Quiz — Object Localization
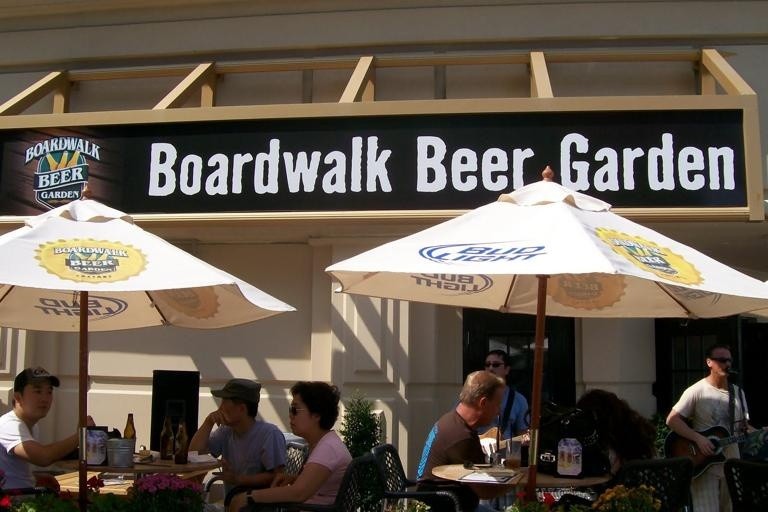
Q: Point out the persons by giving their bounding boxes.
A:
[0,365,94,499]
[475,350,528,462]
[576,389,655,461]
[224,381,352,512]
[414,371,529,512]
[188,377,288,512]
[663,346,757,512]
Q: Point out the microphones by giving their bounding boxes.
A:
[725,367,739,376]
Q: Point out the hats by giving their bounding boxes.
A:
[14,367,60,392]
[211,379,261,402]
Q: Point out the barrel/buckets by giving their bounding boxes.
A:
[105,438,136,468]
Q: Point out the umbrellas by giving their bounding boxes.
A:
[321,166,766,501]
[0,182,296,512]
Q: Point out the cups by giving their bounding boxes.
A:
[505,441,522,470]
[489,452,501,468]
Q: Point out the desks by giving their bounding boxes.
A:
[56,452,225,502]
[427,462,613,512]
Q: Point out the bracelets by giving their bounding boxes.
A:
[245,490,255,506]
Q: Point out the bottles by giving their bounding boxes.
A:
[123,413,136,439]
[175,421,190,464]
[159,411,175,461]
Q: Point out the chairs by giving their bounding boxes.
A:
[0,487,57,512]
[198,437,480,512]
[720,454,767,511]
[614,454,693,511]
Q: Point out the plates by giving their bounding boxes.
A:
[133,456,154,465]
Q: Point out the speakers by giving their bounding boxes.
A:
[150,370,200,453]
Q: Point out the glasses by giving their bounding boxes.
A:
[711,357,732,363]
[289,406,304,414]
[484,361,504,368]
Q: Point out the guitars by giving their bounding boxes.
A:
[666,426,768,479]
[475,426,529,463]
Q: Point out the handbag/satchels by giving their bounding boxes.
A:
[539,409,606,480]
